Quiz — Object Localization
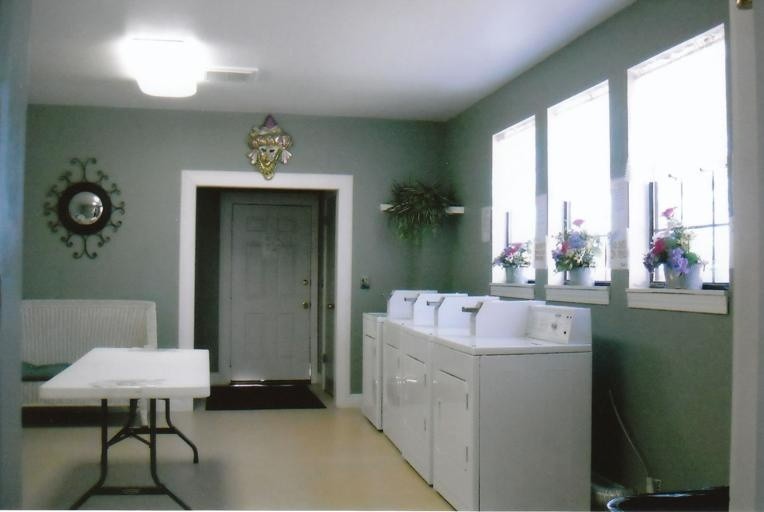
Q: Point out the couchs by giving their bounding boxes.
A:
[21,295,160,430]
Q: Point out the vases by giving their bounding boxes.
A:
[685,263,707,294]
[571,267,596,290]
[664,264,685,289]
[507,268,526,287]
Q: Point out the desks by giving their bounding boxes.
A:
[39,344,210,511]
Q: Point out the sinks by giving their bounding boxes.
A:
[605,488,728,512]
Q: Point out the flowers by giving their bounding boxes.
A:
[492,238,534,266]
[641,205,707,271]
[553,217,600,266]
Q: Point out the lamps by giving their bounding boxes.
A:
[119,37,215,100]
